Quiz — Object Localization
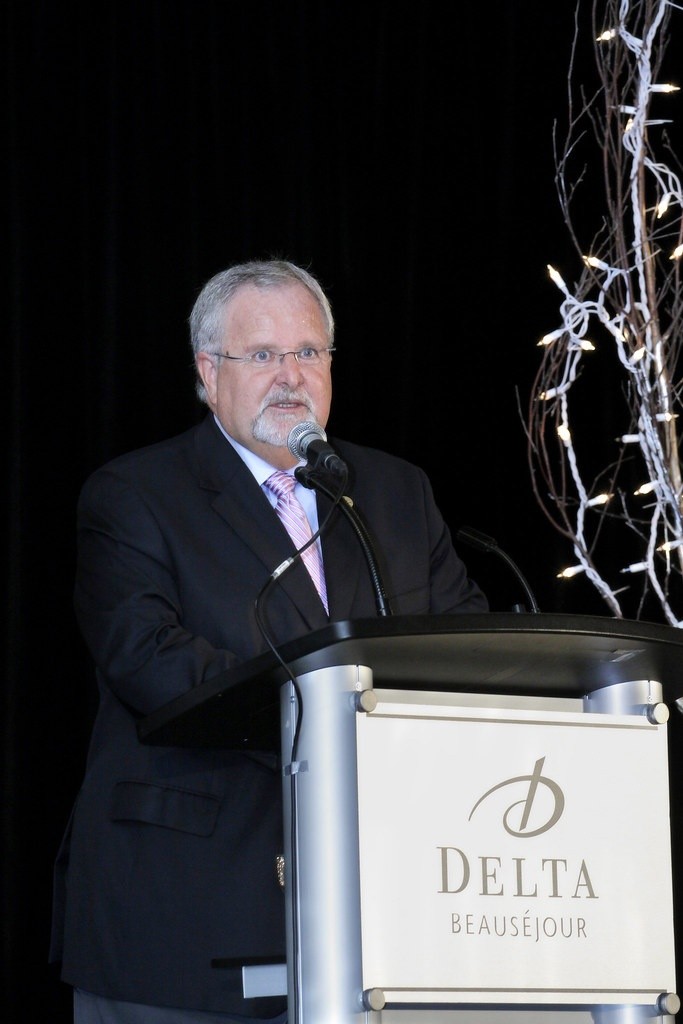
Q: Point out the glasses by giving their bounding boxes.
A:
[214,347,336,367]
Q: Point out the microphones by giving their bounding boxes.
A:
[459,527,539,615]
[287,421,347,478]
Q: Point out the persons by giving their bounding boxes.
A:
[60,260,489,1024]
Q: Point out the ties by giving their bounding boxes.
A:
[264,469,329,615]
